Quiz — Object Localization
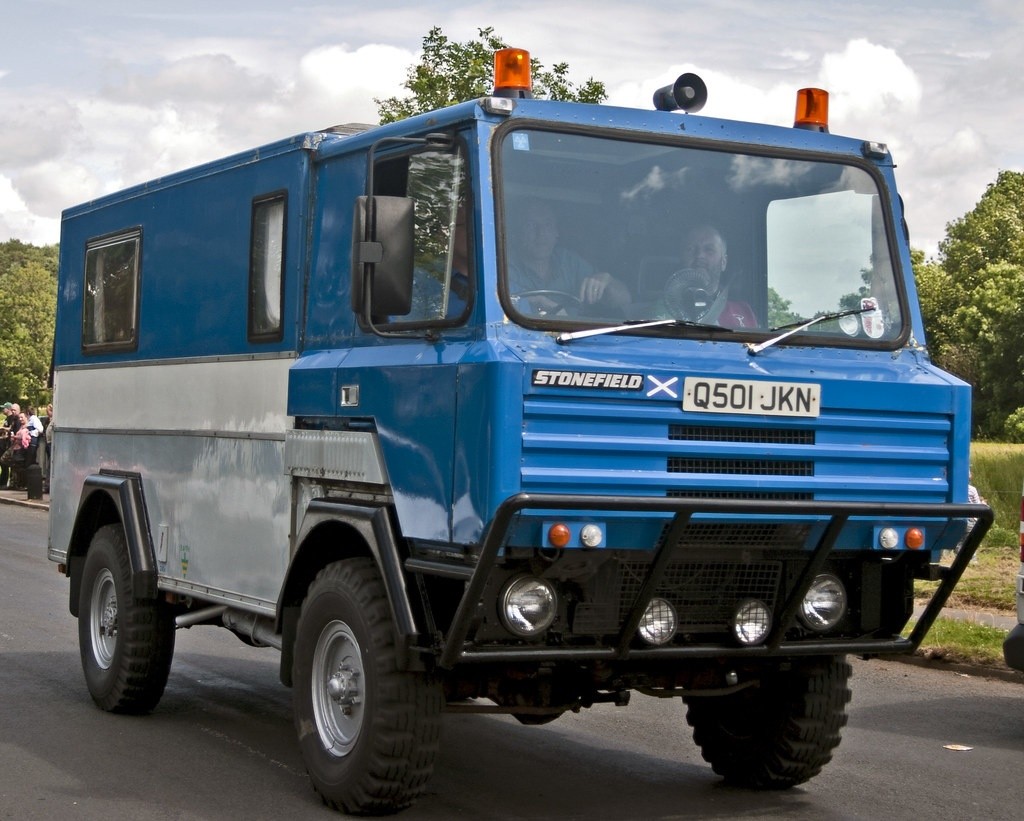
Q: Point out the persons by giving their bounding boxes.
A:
[653,223,762,330]
[388,201,535,320]
[508,198,632,320]
[0,401,53,494]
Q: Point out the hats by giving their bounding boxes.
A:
[0,402,12,410]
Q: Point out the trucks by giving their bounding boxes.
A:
[42,47,996,821]
